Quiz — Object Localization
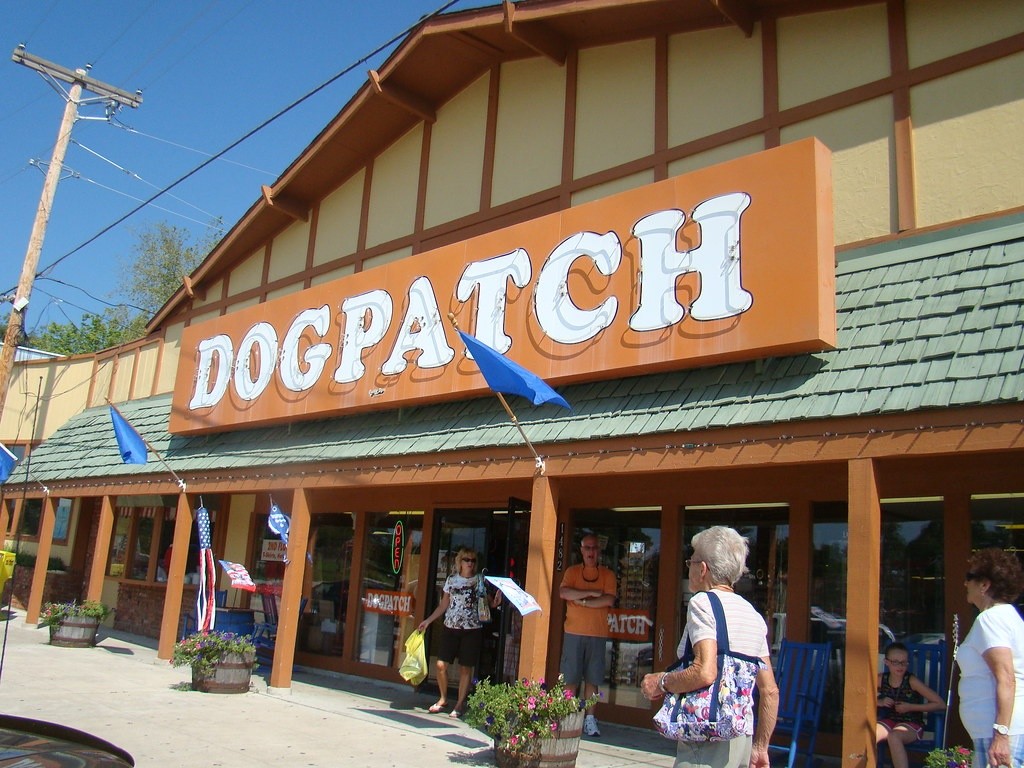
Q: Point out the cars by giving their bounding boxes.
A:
[605,616,656,677]
[806,619,945,693]
[129,538,150,576]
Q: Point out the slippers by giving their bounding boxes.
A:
[449,709,466,718]
[429,703,447,713]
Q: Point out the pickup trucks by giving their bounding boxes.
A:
[631,610,893,714]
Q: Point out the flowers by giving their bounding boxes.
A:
[924,744,975,768]
[169,630,260,679]
[41,598,109,632]
[462,674,604,755]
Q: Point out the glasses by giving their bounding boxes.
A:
[582,569,599,582]
[884,657,910,667]
[966,573,978,581]
[685,560,710,571]
[582,545,598,551]
[462,557,476,563]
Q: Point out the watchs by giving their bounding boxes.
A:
[992,723,1009,735]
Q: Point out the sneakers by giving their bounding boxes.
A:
[583,718,601,736]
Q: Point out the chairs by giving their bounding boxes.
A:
[875,639,948,768]
[248,598,309,674]
[751,637,832,768]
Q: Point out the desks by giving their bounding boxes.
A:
[215,606,269,638]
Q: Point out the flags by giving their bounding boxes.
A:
[109,405,147,464]
[0,442,19,486]
[459,331,572,411]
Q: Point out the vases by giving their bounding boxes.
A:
[493,708,585,768]
[49,616,100,647]
[191,650,256,693]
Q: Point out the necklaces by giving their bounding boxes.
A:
[891,680,902,705]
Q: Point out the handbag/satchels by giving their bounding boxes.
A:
[652,590,766,743]
[478,573,493,623]
[399,628,428,687]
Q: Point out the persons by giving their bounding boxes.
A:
[418,548,503,717]
[640,525,779,768]
[559,535,617,735]
[876,642,946,768]
[956,548,1024,768]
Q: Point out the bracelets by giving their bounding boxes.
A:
[582,598,586,607]
[658,672,670,695]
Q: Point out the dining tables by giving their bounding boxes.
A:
[181,590,228,641]
